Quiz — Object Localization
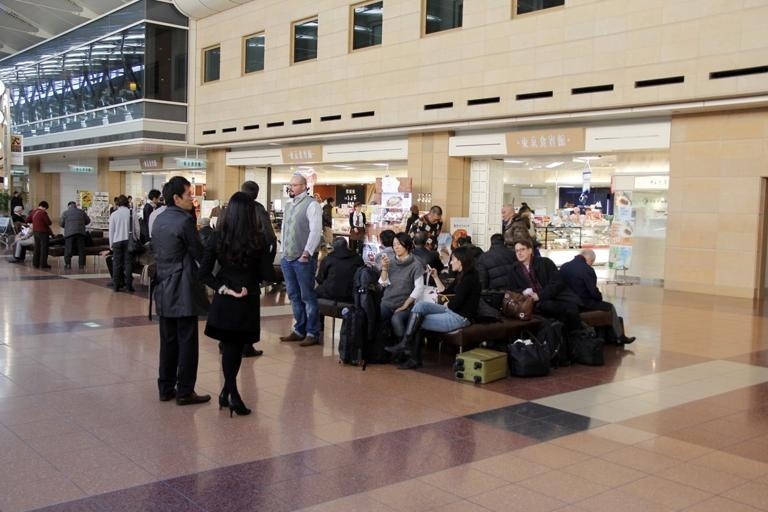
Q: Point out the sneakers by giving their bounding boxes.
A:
[279,331,305,341]
[298,335,319,347]
[243,349,264,358]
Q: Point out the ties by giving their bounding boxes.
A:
[356,215,360,227]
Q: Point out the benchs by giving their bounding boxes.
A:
[315,294,613,357]
[0,228,110,278]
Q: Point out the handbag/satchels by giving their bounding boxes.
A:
[570,328,606,367]
[438,293,457,304]
[477,323,510,351]
[534,321,570,370]
[502,288,535,320]
[422,284,440,303]
[127,232,147,257]
[507,342,549,380]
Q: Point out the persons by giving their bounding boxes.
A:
[58,201,89,270]
[148,175,211,406]
[198,191,274,419]
[312,192,635,372]
[196,205,225,320]
[218,181,276,359]
[10,190,51,270]
[278,175,322,347]
[105,188,163,293]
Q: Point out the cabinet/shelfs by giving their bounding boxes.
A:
[535,226,609,267]
[377,205,408,232]
[332,217,351,248]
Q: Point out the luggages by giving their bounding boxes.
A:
[454,348,507,385]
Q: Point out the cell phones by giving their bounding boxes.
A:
[425,264,432,272]
[383,254,388,259]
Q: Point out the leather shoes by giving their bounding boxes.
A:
[159,389,176,401]
[176,391,211,406]
[616,334,637,345]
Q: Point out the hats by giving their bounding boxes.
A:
[68,201,76,206]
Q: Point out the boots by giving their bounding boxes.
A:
[397,328,425,370]
[384,310,425,353]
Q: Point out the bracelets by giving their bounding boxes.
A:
[301,254,309,259]
[221,287,227,294]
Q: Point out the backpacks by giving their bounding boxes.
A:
[338,306,367,365]
[353,260,381,317]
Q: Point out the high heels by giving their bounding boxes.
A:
[218,391,229,411]
[229,401,252,418]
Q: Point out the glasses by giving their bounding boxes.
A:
[287,183,304,188]
[515,247,526,253]
